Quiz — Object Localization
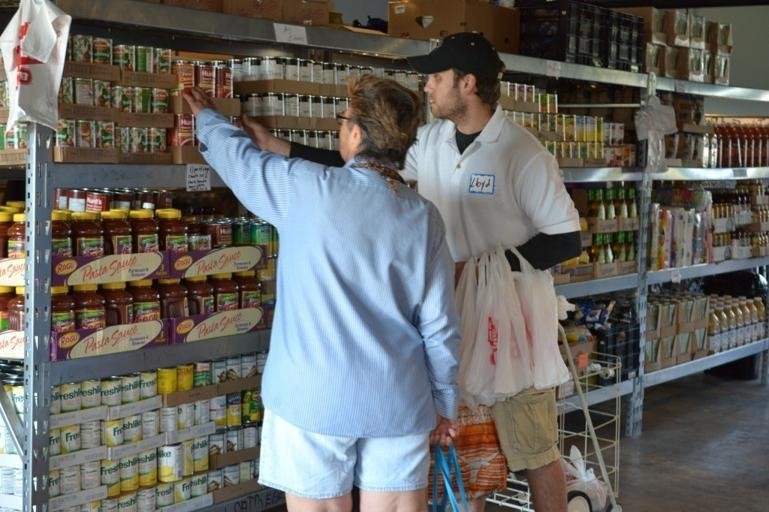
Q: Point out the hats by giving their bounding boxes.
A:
[407,32,499,79]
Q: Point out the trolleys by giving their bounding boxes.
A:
[486,320,624,512]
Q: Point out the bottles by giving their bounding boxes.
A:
[714,122,769,168]
[579,181,769,355]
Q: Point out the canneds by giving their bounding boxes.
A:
[1,181,277,335]
[0,33,638,169]
[49,350,268,512]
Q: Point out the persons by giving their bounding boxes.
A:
[182,75,460,512]
[241,32,582,512]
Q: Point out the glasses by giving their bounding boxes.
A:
[336,111,368,131]
